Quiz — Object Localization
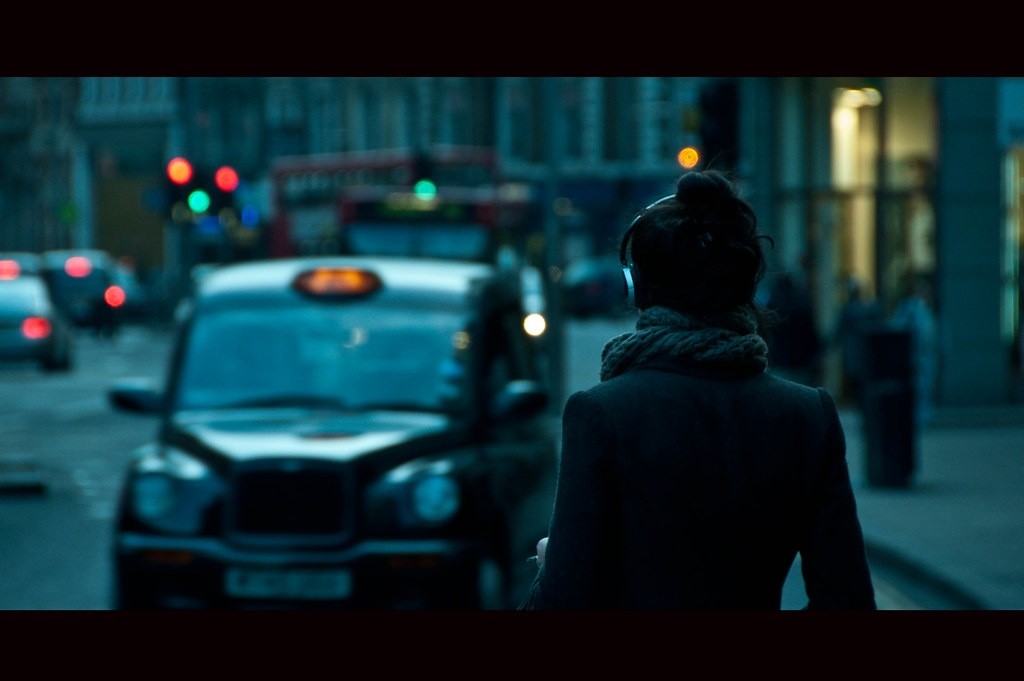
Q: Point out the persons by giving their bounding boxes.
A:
[530,169,878,612]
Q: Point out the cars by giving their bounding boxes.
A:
[0,233,134,375]
[105,250,562,629]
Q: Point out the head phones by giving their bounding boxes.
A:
[620,192,767,310]
